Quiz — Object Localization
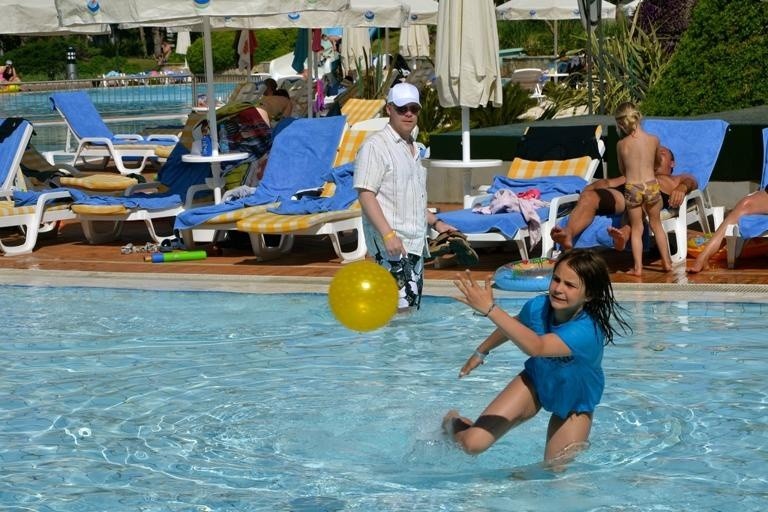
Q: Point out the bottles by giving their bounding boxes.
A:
[201,135,212,156]
[219,124,229,153]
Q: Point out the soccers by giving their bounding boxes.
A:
[329,261,400,331]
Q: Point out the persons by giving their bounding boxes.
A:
[315,45,329,68]
[354,82,462,310]
[1,60,21,84]
[551,97,768,276]
[155,42,175,66]
[379,54,413,91]
[322,33,342,54]
[255,78,295,126]
[440,251,608,471]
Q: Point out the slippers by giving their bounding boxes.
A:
[159,238,184,252]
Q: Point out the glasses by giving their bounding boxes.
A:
[391,103,419,115]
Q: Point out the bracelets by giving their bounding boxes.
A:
[382,231,399,241]
[473,349,490,359]
[470,304,499,317]
[432,218,443,228]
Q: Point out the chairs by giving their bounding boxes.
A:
[551,118,733,266]
[427,124,607,267]
[721,127,767,268]
[511,69,545,109]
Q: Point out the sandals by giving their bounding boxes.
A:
[450,230,478,268]
[428,231,452,257]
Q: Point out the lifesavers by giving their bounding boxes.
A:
[493,257,556,292]
[0,66,20,94]
[687,227,727,263]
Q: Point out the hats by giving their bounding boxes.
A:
[387,82,422,107]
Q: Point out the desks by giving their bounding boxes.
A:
[545,73,568,84]
